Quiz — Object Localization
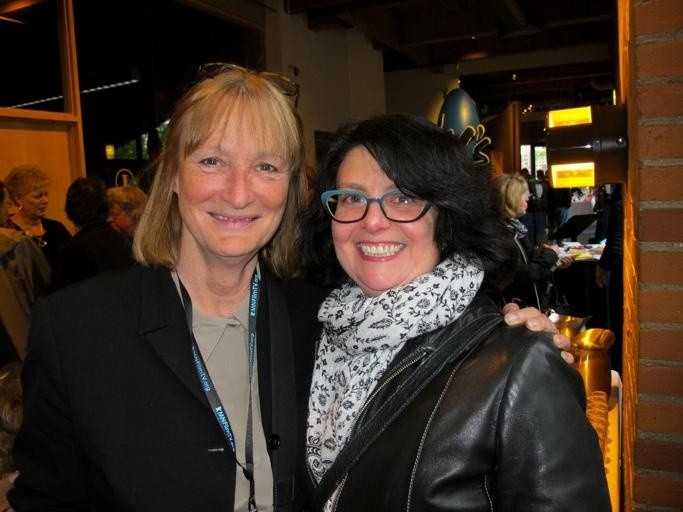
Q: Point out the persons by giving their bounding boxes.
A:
[0,167,150,361]
[278,110,616,511]
[13,57,576,512]
[493,168,605,316]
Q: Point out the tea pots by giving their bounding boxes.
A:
[544,307,615,401]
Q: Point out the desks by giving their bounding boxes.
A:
[602,370,624,512]
[548,241,607,325]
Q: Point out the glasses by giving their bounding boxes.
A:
[321,188,435,222]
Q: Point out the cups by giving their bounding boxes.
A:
[586,390,607,470]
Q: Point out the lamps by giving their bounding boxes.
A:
[545,101,628,191]
